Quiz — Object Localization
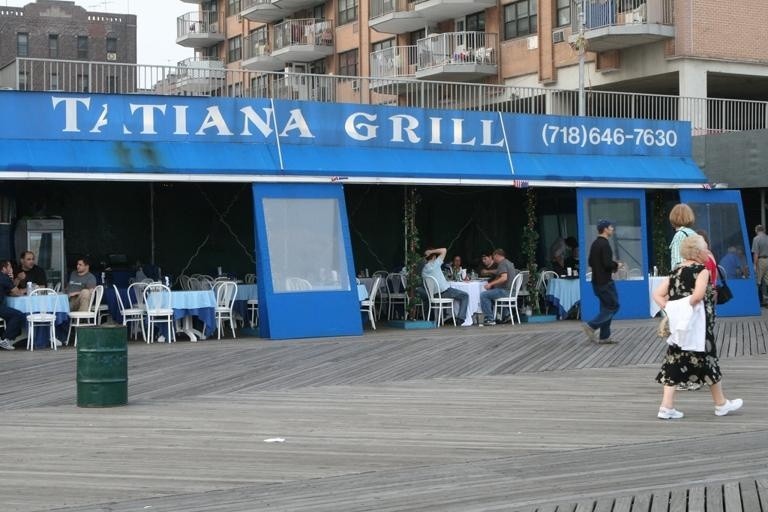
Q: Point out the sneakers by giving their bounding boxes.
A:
[581,324,596,343]
[656,405,685,420]
[484,320,496,326]
[501,314,513,324]
[713,396,745,416]
[0,337,15,350]
[598,336,616,345]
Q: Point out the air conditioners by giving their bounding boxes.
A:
[210,24,217,32]
[106,53,116,61]
[552,29,566,44]
[235,14,242,23]
[352,80,360,90]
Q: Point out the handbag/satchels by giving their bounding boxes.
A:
[716,282,733,305]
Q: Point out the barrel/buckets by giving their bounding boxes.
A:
[75,323,129,409]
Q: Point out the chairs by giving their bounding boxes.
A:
[625,3,647,23]
[474,46,493,65]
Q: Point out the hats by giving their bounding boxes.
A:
[597,219,616,230]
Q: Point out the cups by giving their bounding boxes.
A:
[47,283,53,294]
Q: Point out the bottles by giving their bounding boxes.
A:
[444,266,479,280]
[26,281,45,296]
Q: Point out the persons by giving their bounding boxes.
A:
[0,258,21,350]
[15,250,46,294]
[669,204,706,269]
[653,236,743,419]
[62,257,97,311]
[583,219,623,344]
[417,237,578,326]
[718,222,767,308]
